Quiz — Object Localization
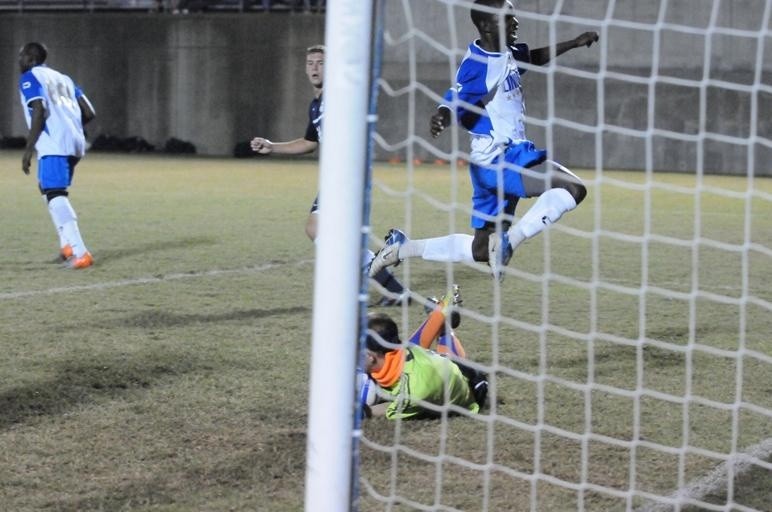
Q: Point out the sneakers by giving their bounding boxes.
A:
[50,244,93,268]
[368,229,406,279]
[488,230,512,280]
[368,289,412,307]
[424,285,461,336]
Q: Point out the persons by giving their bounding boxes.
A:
[354,284,489,421]
[367,0,599,283]
[249,45,411,309]
[16,41,97,270]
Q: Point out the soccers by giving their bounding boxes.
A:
[358,369,381,407]
